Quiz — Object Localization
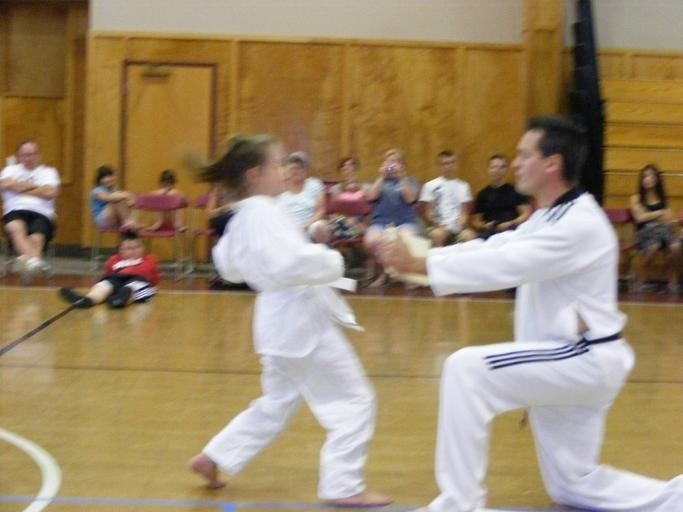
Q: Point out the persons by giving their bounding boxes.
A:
[0,140,61,279]
[147,170,184,230]
[626,163,682,297]
[86,165,143,237]
[206,148,535,299]
[57,228,161,311]
[179,130,390,507]
[370,114,682,511]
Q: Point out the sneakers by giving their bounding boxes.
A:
[8,257,54,283]
[61,288,91,308]
[108,287,132,308]
